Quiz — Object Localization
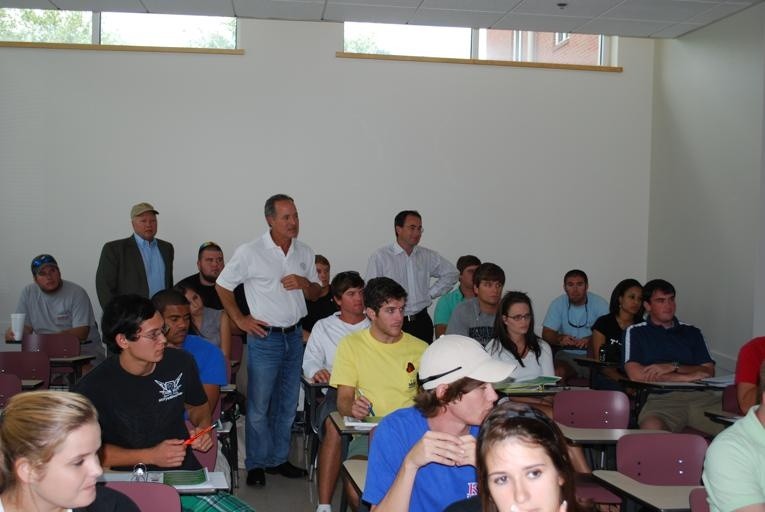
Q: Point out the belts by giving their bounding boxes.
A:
[257,320,302,333]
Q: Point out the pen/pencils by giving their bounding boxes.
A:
[356,389,375,417]
[184,424,218,444]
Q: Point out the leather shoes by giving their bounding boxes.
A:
[265,460,308,478]
[247,468,265,487]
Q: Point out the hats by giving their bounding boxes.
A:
[130,201,159,219]
[418,334,518,391]
[30,254,58,274]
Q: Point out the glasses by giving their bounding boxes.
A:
[403,223,424,233]
[139,325,170,340]
[417,367,462,388]
[568,314,588,328]
[507,313,531,321]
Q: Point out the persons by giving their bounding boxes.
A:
[301,255,764,512]
[0,242,249,511]
[362,208,462,345]
[95,201,174,359]
[215,194,324,489]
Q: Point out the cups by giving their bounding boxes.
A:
[10,313,26,341]
